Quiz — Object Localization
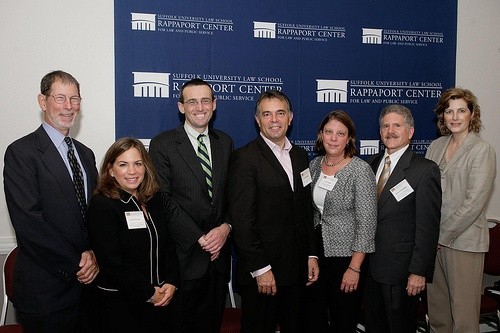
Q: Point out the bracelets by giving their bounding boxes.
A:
[349,265,360,273]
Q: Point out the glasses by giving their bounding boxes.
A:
[182,97,215,106]
[46,93,84,106]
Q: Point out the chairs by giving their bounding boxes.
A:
[0,246,22,333]
[478,218,500,333]
[220,257,242,333]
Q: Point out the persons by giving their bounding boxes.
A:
[228,91,320,333]
[309,109,377,333]
[150,79,235,333]
[85,136,182,333]
[425,89,497,333]
[362,104,440,333]
[3,70,100,333]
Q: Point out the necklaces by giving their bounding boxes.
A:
[324,153,345,167]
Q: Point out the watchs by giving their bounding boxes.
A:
[224,222,232,231]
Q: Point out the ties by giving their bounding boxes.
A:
[65,136,88,220]
[197,135,212,206]
[377,156,391,203]
